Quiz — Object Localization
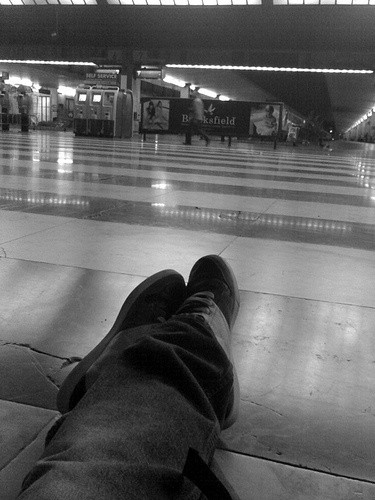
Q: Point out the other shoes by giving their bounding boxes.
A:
[56,268,187,415]
[178,254,242,431]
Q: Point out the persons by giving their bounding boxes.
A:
[183,91,212,147]
[16,254,244,500]
[256,106,278,138]
[142,100,171,131]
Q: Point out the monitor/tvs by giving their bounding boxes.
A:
[92,93,101,103]
[78,93,87,102]
